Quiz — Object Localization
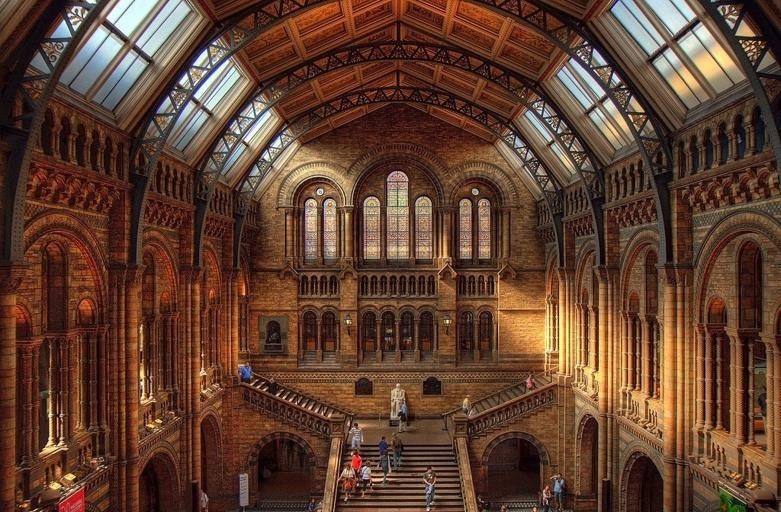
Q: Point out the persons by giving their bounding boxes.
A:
[423,465,437,512]
[399,401,411,427]
[526,373,538,391]
[200,489,210,511]
[283,439,296,465]
[550,472,565,511]
[757,390,768,436]
[462,394,473,416]
[397,409,407,433]
[334,422,406,501]
[241,361,253,384]
[308,499,316,512]
[390,382,407,417]
[265,378,278,395]
[476,484,553,511]
[469,405,478,434]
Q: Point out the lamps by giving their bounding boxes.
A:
[443,314,453,335]
[344,314,353,336]
